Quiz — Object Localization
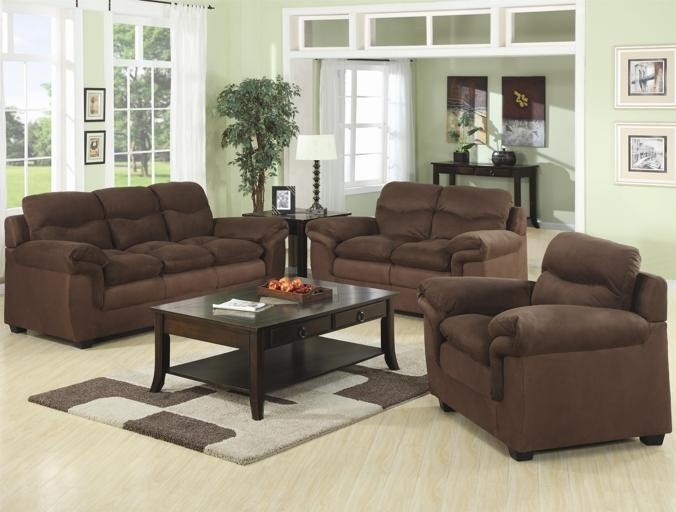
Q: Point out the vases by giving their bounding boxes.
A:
[492,151,516,165]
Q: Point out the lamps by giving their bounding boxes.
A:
[296,135,338,215]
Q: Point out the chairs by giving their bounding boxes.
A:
[417,270,676,462]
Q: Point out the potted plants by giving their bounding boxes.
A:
[447,110,482,163]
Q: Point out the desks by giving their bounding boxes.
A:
[431,162,540,229]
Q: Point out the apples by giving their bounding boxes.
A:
[268,276,303,292]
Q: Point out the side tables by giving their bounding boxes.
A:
[242,208,352,278]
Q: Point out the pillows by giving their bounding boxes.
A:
[430,185,513,240]
[375,181,444,240]
[21,182,214,251]
[530,231,641,311]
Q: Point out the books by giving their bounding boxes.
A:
[213,309,257,319]
[213,298,272,313]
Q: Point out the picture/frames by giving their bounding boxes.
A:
[614,44,675,108]
[84,88,106,122]
[84,131,106,165]
[272,186,295,215]
[615,122,675,187]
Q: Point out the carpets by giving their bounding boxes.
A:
[27,329,430,467]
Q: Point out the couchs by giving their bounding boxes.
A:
[3,182,290,347]
[305,182,528,315]
